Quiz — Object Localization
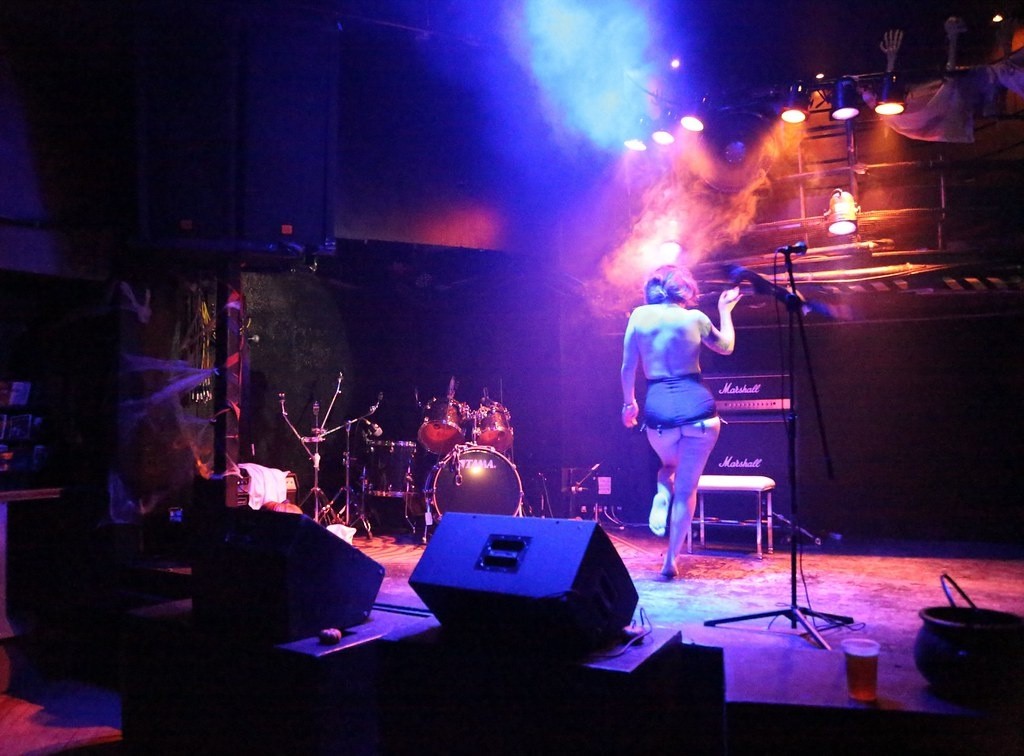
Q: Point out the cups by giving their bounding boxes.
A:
[841,637,881,702]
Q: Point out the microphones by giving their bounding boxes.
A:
[777,242,807,256]
[571,486,588,492]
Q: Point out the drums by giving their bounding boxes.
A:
[361,439,417,498]
[473,402,513,454]
[424,444,523,524]
[417,396,467,457]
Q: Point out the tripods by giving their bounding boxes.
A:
[704,254,854,650]
[298,401,372,539]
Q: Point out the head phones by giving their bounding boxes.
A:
[646,274,674,306]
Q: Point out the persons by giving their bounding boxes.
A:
[424,368,472,481]
[620,262,743,576]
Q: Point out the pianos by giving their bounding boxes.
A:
[700,371,821,546]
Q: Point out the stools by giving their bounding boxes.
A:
[686,474,776,559]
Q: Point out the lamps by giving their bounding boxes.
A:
[825,187,862,235]
[622,72,910,150]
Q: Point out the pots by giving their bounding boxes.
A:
[913,572,1024,710]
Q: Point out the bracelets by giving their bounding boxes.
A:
[624,398,636,409]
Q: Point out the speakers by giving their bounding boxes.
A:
[192,509,387,646]
[407,510,638,646]
[691,415,800,532]
